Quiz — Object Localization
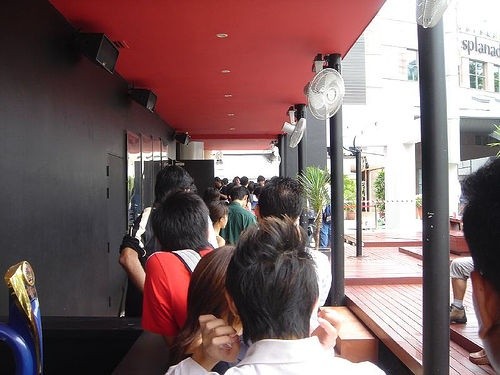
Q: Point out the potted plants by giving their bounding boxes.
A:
[343,174,356,220]
[373,168,385,221]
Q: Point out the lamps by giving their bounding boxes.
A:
[76,32,191,145]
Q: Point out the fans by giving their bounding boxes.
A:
[281,106,306,148]
[303,53,345,120]
[266,143,281,164]
[416,0,451,29]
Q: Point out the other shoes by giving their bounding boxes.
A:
[469,349,490,365]
[450,303,467,323]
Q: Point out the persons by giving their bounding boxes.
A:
[141,189,215,350]
[449,257,475,324]
[205,175,270,247]
[460,156,500,375]
[158,246,252,375]
[319,183,332,247]
[255,175,332,307]
[166,217,386,375]
[118,165,194,316]
[207,201,230,247]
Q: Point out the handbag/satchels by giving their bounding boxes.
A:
[323,211,332,224]
